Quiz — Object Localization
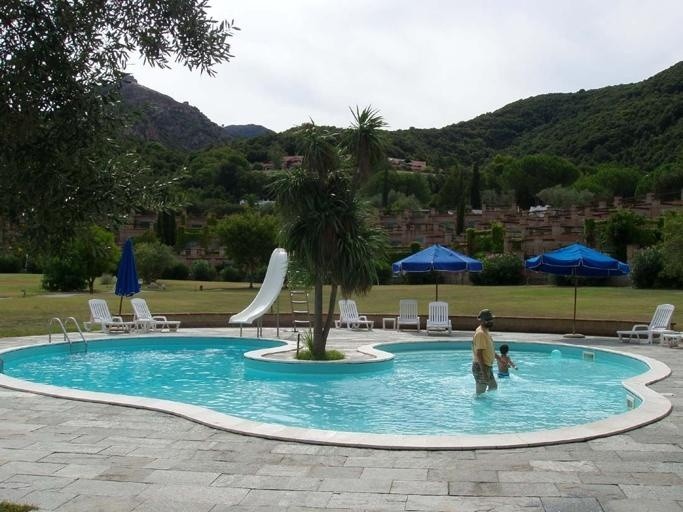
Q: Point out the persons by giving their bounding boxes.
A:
[498,344,518,378]
[472,309,507,394]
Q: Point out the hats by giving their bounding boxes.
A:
[478,308,495,321]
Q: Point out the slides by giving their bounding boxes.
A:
[228,248,295,324]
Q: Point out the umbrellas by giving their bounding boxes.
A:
[526,242,631,334]
[114,237,141,314]
[392,243,483,302]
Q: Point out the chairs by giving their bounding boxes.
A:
[131,298,181,333]
[83,297,150,336]
[426,302,452,336]
[335,300,374,332]
[396,299,420,333]
[662,333,683,349]
[616,304,675,344]
